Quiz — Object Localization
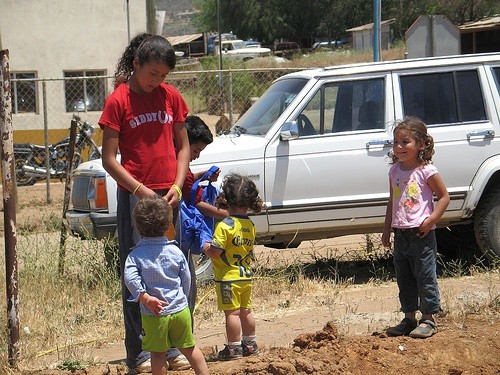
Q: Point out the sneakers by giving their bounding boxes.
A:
[241,339,260,357]
[167,353,192,371]
[217,343,243,361]
[129,358,169,373]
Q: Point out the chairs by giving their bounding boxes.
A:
[357,102,380,128]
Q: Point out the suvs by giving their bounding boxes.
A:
[64,50,500,271]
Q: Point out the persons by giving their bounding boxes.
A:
[382,117,450,338]
[174,115,262,360]
[123,196,208,375]
[97,32,209,373]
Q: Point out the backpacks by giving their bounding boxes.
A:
[190,165,222,232]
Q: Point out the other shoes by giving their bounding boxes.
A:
[408,318,438,338]
[387,318,419,336]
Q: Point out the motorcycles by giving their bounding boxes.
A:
[13,106,119,187]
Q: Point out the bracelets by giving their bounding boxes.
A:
[171,184,183,200]
[133,182,143,195]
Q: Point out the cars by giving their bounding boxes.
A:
[71,93,93,112]
[207,30,343,82]
